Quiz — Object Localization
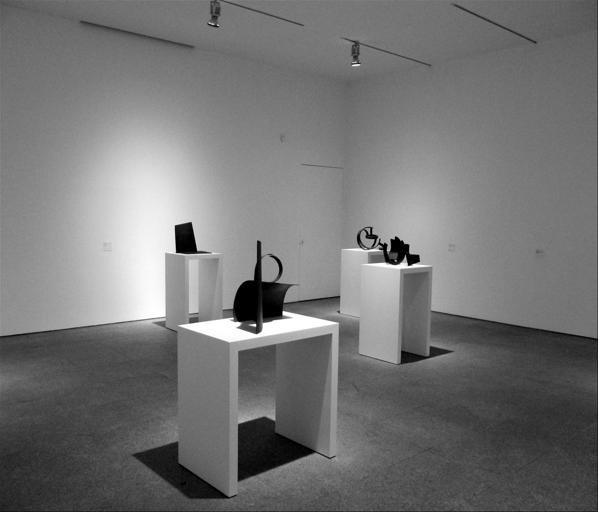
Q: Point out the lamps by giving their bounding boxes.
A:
[205,0,222,29]
[350,41,362,68]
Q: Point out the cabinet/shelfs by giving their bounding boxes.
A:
[163,250,224,331]
[358,262,435,366]
[338,248,390,317]
[175,308,340,499]
